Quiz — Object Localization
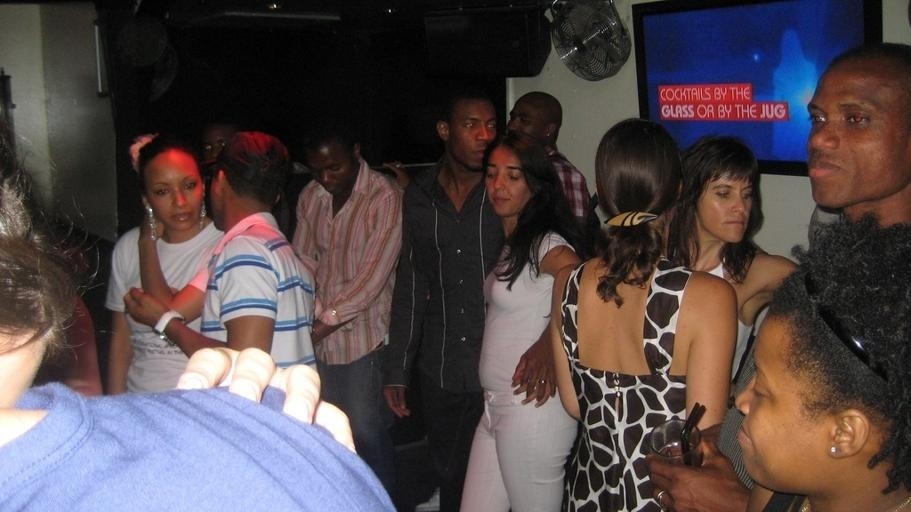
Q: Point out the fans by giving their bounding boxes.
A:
[551,0,632,82]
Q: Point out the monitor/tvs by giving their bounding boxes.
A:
[631,0,884,178]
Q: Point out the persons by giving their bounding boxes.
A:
[3,40,911,511]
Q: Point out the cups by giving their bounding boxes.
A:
[648,418,704,468]
